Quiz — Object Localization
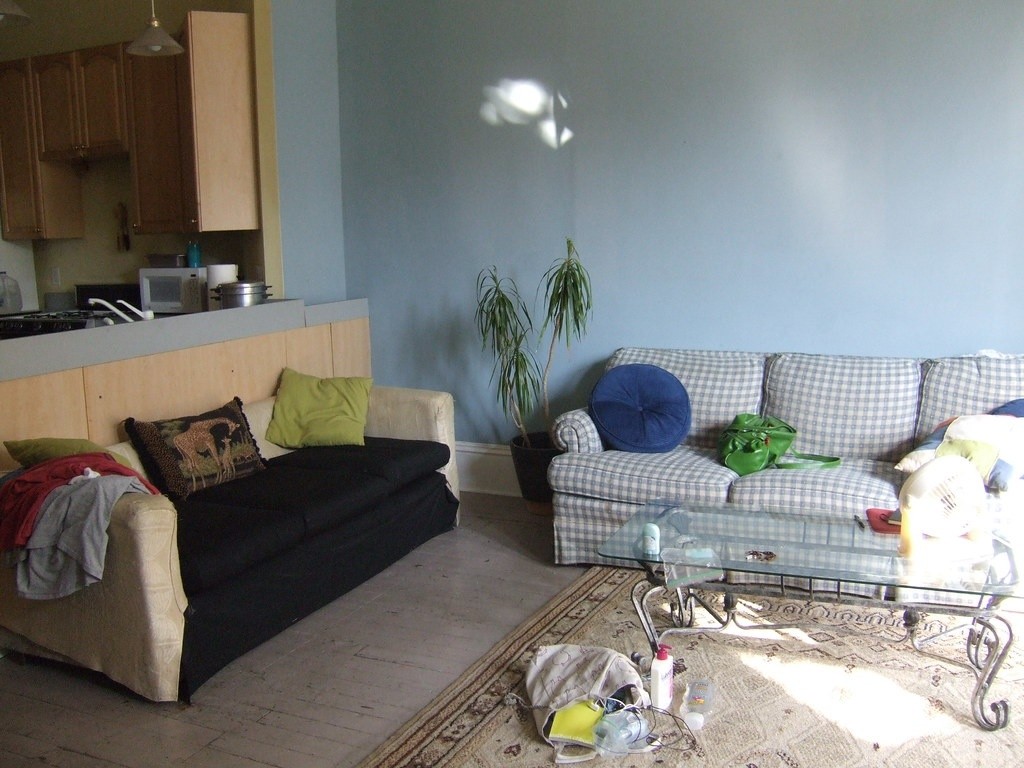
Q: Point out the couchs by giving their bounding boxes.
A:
[1,383,459,702]
[547,345,1024,602]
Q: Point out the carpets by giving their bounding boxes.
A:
[353,568,1024,768]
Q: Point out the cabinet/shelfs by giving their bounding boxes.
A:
[34,42,125,161]
[129,35,187,236]
[0,58,85,241]
[175,11,256,235]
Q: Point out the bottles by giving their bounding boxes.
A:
[680,680,712,731]
[0,271,23,314]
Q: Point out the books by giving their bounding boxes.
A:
[548,699,605,764]
[866,509,902,534]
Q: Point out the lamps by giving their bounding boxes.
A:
[126,0,186,57]
[0,0,34,27]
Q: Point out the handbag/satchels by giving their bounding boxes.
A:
[718,413,842,477]
[524,643,664,763]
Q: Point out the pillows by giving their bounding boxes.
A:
[587,363,693,452]
[2,438,130,473]
[266,368,374,447]
[124,398,267,505]
[894,412,1024,491]
[988,399,1024,416]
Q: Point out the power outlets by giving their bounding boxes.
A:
[51,267,61,286]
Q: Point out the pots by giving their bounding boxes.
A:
[210,274,274,309]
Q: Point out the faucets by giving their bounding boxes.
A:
[88,298,154,322]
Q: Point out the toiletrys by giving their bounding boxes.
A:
[679,681,715,730]
[651,643,674,711]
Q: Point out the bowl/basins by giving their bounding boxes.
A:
[144,254,187,269]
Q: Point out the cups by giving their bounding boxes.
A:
[207,264,239,311]
[187,244,201,268]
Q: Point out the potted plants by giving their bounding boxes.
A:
[475,235,593,516]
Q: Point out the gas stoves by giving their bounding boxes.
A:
[0,283,143,340]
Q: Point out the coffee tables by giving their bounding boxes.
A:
[599,498,1024,732]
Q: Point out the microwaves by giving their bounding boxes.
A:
[139,267,207,313]
